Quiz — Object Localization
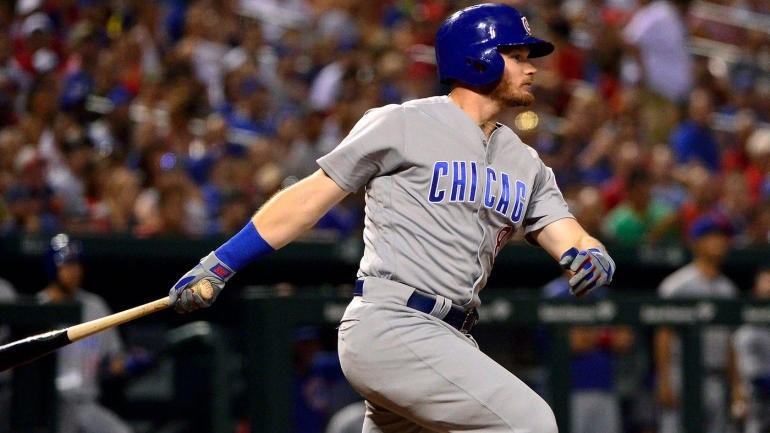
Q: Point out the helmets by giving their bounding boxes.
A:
[435,2,557,95]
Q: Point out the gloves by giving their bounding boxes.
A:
[559,246,615,301]
[167,250,236,313]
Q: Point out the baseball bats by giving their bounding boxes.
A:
[0,280,216,372]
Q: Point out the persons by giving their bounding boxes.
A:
[169,3,616,433]
[0,1,770,433]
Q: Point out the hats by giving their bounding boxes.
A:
[51,236,82,265]
[691,211,733,239]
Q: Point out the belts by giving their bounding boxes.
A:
[354,281,478,336]
